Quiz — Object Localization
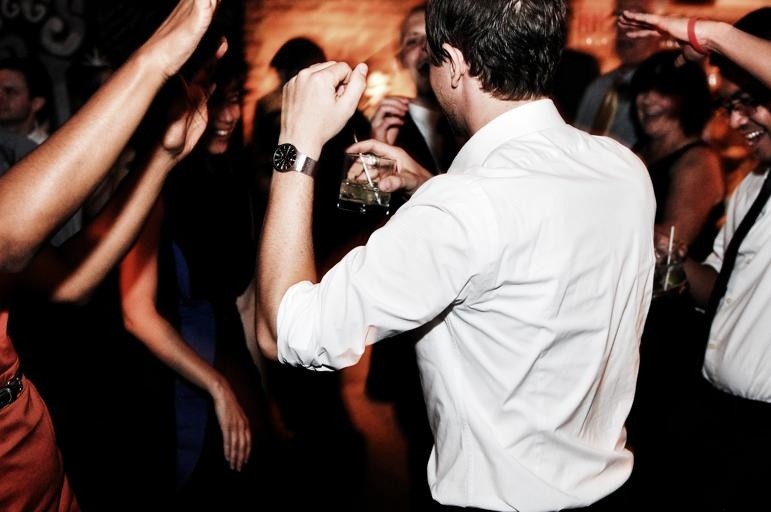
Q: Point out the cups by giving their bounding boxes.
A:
[337,153,396,219]
[652,240,687,299]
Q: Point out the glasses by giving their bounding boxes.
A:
[720,100,757,118]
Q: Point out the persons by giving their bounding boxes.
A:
[2,1,771,511]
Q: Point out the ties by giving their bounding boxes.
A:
[701,168,771,339]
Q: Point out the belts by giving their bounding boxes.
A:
[0,369,22,408]
